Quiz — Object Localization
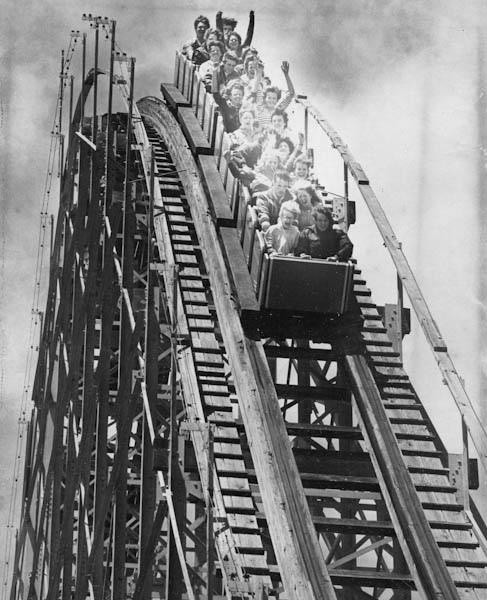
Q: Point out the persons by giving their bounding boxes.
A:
[180,9,350,263]
